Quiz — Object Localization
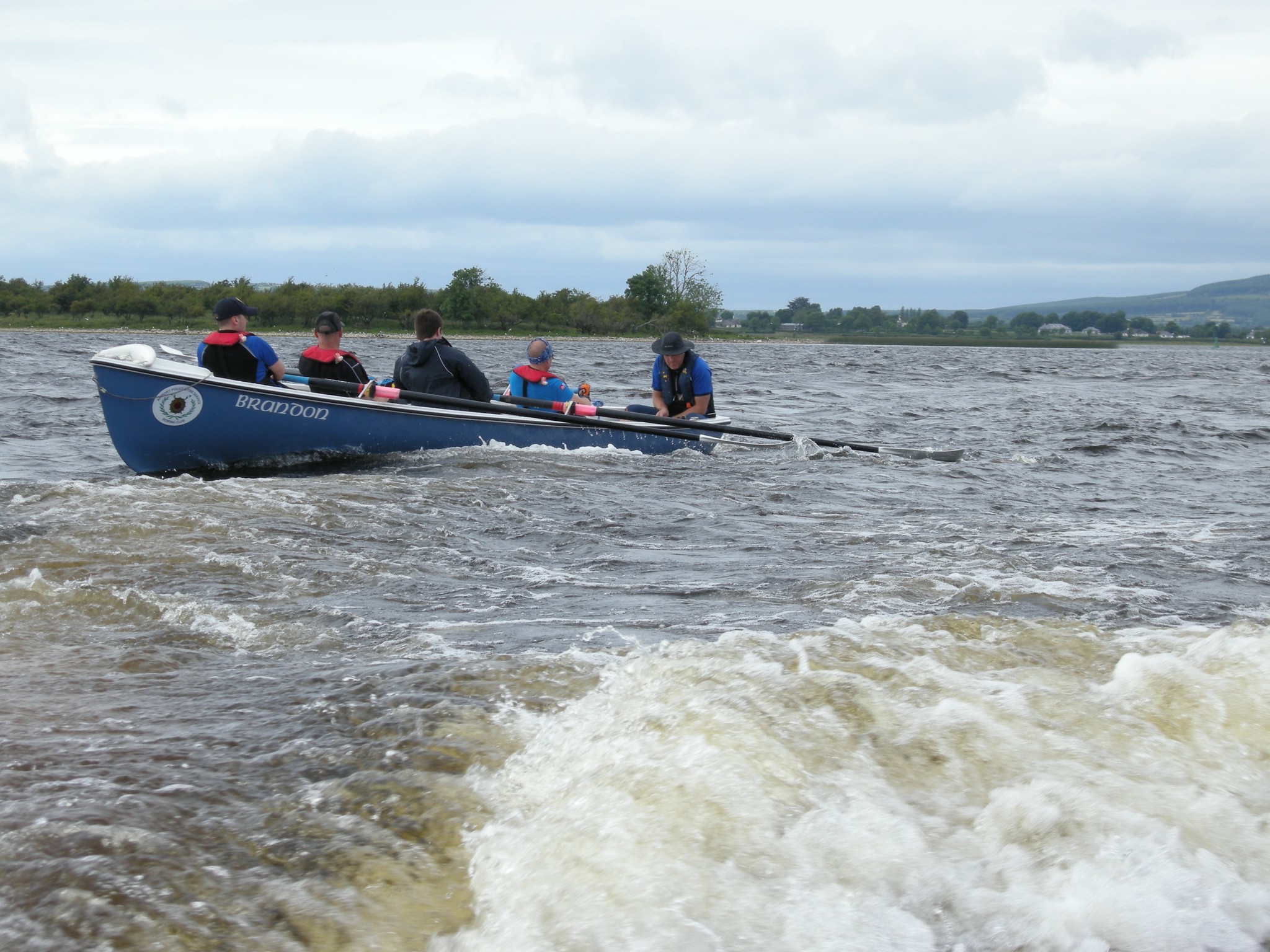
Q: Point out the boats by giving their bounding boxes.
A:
[88,342,733,483]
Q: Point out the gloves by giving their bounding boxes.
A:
[578,384,592,401]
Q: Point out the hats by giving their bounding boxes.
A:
[315,311,344,334]
[651,332,695,355]
[213,297,257,320]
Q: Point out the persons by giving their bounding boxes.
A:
[394,310,500,414]
[298,312,407,404]
[625,332,716,419]
[509,338,591,418]
[197,297,296,390]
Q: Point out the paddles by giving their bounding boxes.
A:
[491,395,965,462]
[160,344,302,376]
[282,373,792,448]
